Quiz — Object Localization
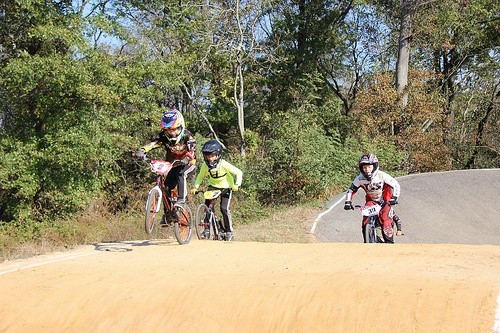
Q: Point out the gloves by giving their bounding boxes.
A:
[181,156,190,166]
[232,184,241,192]
[344,201,354,210]
[388,196,397,205]
[190,186,200,196]
[135,149,145,160]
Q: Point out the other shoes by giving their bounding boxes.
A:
[200,229,209,239]
[226,234,234,241]
[174,199,184,209]
[160,215,165,224]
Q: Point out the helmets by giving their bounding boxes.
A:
[359,153,379,181]
[202,140,222,168]
[162,109,185,145]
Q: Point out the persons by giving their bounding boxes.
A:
[135,109,197,227]
[190,140,243,242]
[345,153,401,244]
